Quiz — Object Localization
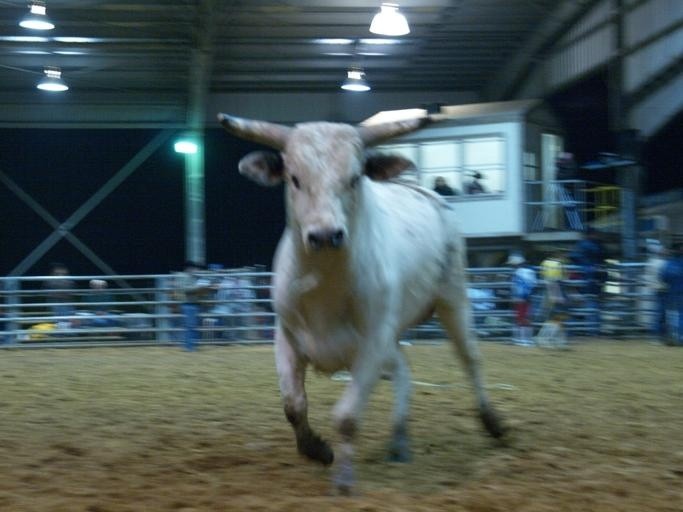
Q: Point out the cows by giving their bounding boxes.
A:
[214,109,508,469]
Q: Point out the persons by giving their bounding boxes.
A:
[16,259,275,353]
[468,226,683,350]
[433,177,456,196]
[469,173,485,193]
[554,152,581,191]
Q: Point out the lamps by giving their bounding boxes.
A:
[18,1,55,32]
[37,65,70,92]
[369,1,410,39]
[340,69,371,95]
